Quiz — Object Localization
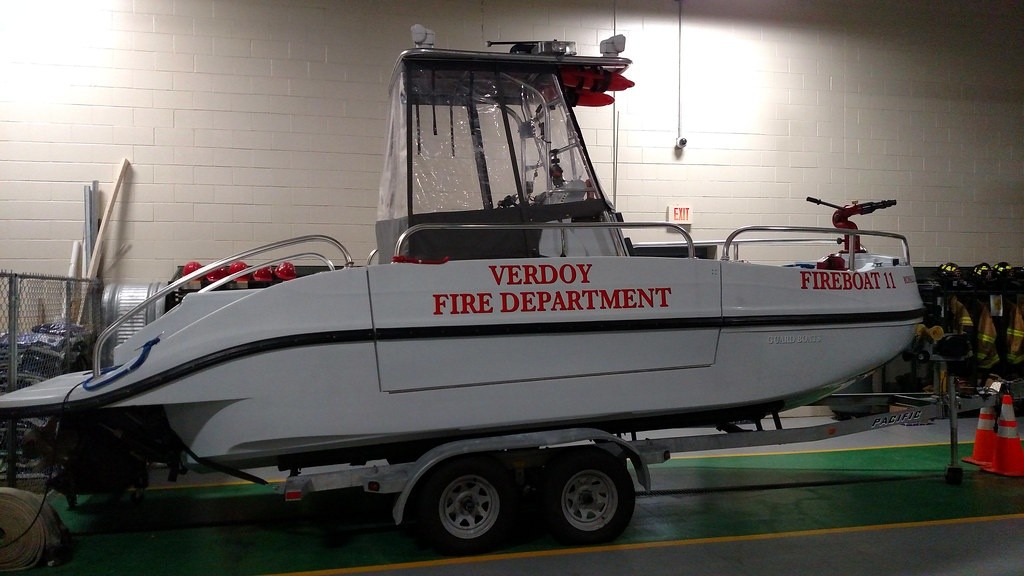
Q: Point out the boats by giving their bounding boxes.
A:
[0,23,925,464]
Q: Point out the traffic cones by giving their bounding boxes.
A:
[961,406,997,466]
[982,395,1024,477]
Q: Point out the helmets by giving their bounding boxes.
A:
[973,262,993,279]
[1009,267,1024,278]
[936,262,963,280]
[993,261,1013,278]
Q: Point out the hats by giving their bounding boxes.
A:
[274,262,296,280]
[253,266,274,282]
[205,266,228,284]
[228,262,252,281]
[182,261,205,281]
